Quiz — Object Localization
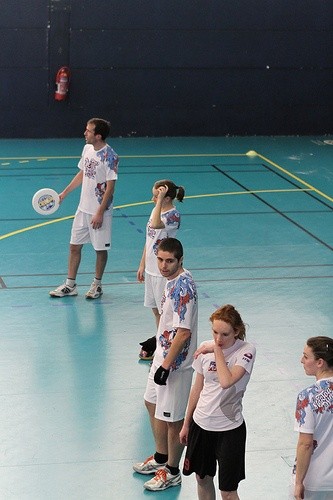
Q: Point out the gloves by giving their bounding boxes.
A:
[138,336,156,355]
[152,366,170,386]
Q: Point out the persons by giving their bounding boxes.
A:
[137,180,185,360]
[132,238,198,491]
[294,336,333,500]
[179,304,256,500]
[49,118,119,300]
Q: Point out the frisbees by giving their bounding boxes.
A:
[32,188,60,216]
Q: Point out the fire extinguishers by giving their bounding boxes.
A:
[53,65,72,102]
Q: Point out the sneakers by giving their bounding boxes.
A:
[132,454,167,474]
[84,283,102,298]
[143,466,182,490]
[48,284,77,297]
